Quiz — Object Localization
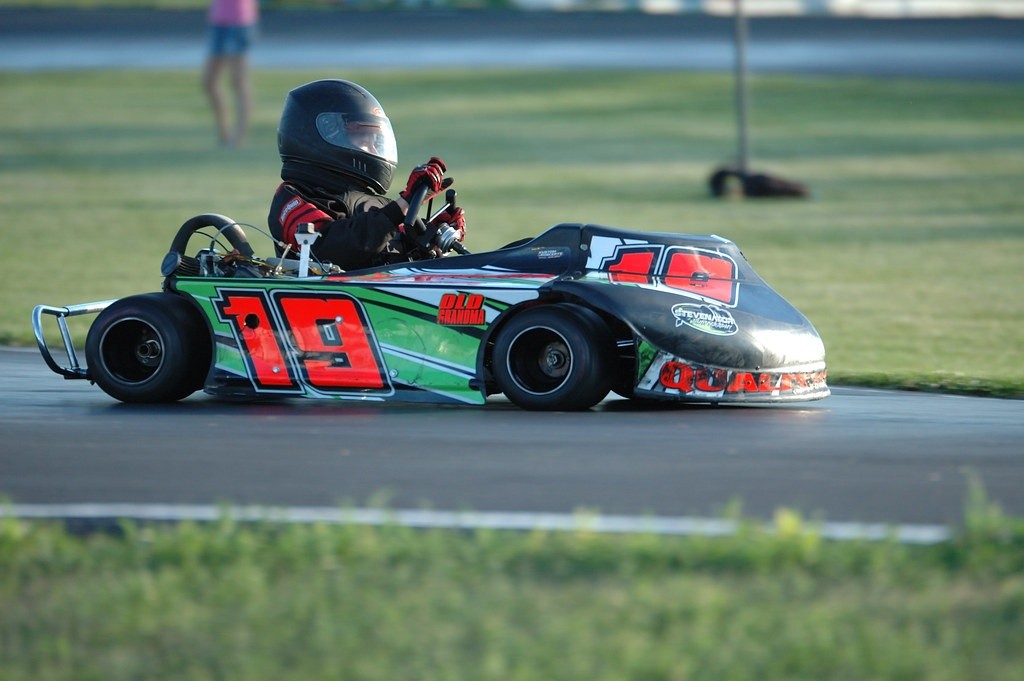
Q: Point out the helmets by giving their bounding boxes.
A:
[276,79,398,220]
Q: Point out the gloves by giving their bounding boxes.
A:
[430,207,466,243]
[399,157,454,207]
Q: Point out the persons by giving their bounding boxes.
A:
[267,79,466,269]
[201,0,259,148]
